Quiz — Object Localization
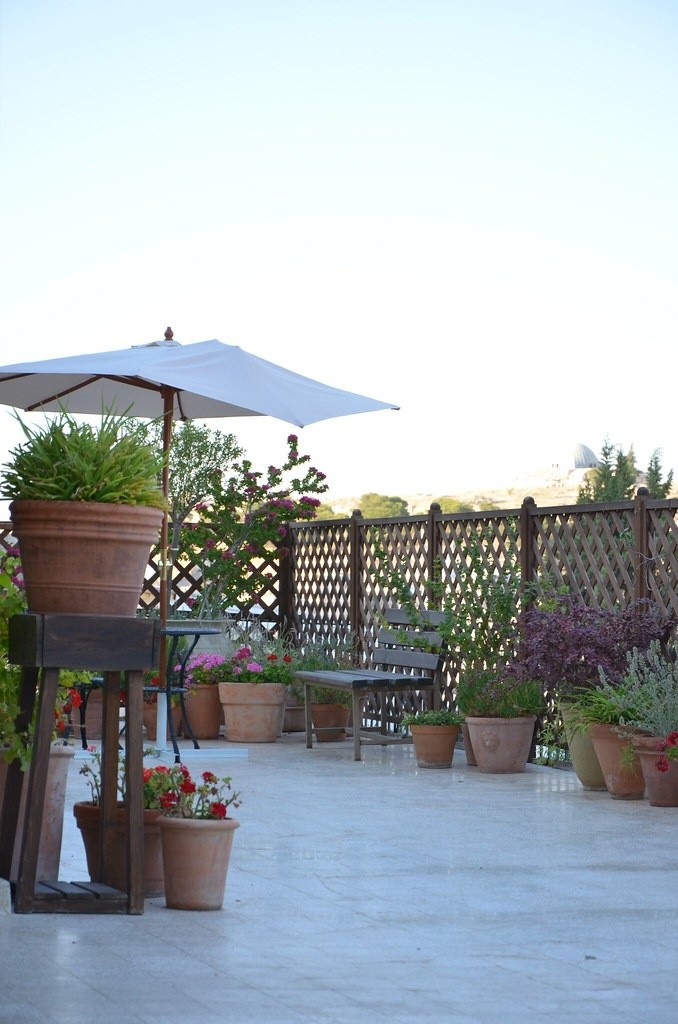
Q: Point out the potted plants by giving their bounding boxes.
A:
[455,668,543,774]
[366,517,539,766]
[142,669,183,741]
[173,651,223,738]
[282,685,306,732]
[348,695,369,728]
[0,593,93,883]
[0,394,179,618]
[597,638,678,807]
[400,709,463,769]
[295,651,352,741]
[218,648,292,742]
[153,762,242,911]
[57,670,103,739]
[502,589,674,792]
[568,685,645,800]
[72,742,178,898]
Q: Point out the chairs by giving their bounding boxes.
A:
[55,677,123,752]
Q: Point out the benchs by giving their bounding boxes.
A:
[294,608,453,760]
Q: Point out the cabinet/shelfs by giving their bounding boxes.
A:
[9,613,163,913]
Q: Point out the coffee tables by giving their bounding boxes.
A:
[119,625,222,764]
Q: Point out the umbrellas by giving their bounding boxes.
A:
[0,325,402,693]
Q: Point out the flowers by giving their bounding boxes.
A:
[143,763,241,820]
[79,745,156,807]
[174,652,227,698]
[181,435,329,609]
[54,688,83,732]
[656,730,678,772]
[0,545,25,599]
[220,646,292,683]
[118,667,160,704]
